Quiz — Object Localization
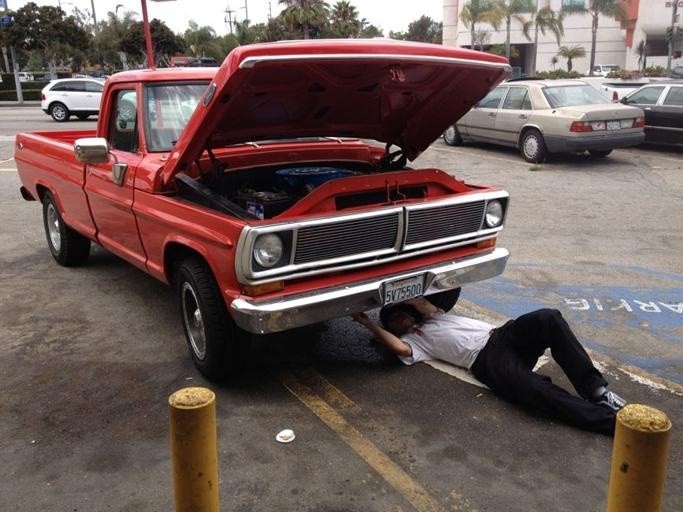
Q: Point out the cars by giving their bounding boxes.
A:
[584,62,682,158]
[439,78,647,165]
[15,70,109,83]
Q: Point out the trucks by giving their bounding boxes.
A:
[168,54,219,67]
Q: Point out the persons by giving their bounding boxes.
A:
[349,294,624,437]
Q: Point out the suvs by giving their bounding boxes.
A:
[39,77,159,123]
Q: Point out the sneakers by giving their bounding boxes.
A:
[589,391,627,412]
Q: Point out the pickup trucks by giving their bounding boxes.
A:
[12,36,517,382]
